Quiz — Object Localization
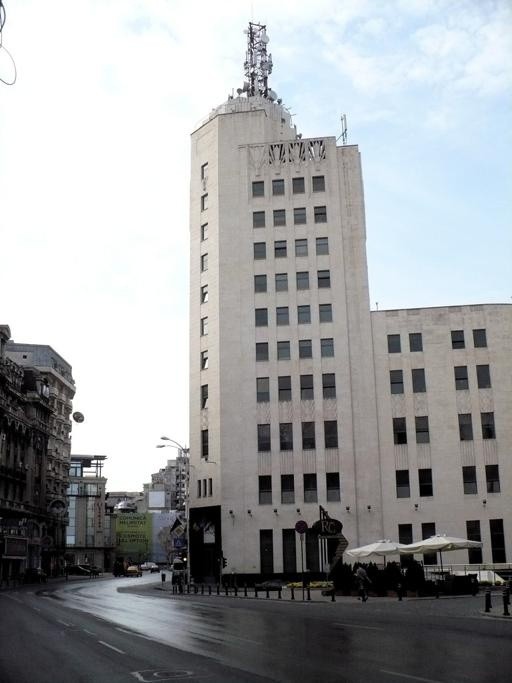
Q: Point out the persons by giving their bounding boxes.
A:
[351,562,372,601]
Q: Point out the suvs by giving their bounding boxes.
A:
[24,567,48,583]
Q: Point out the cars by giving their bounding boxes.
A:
[66,563,104,575]
[112,556,160,578]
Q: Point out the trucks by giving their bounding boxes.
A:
[168,556,190,585]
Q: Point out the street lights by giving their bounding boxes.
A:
[155,435,193,584]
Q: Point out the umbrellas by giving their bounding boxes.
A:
[345,538,406,568]
[397,533,482,580]
